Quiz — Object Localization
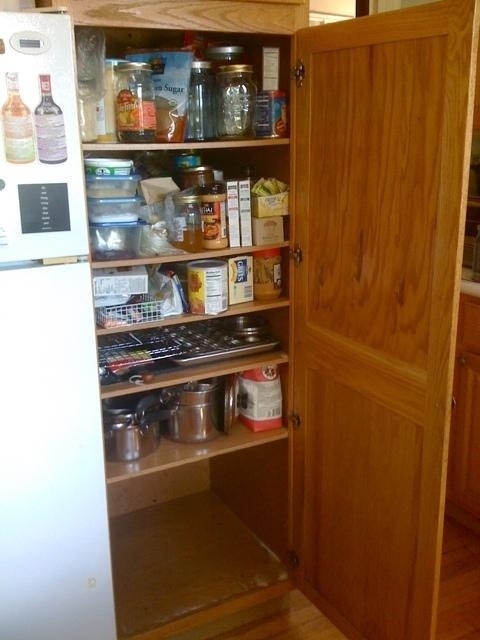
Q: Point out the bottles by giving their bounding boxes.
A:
[0,70,35,166]
[186,61,217,139]
[217,63,258,140]
[207,45,247,63]
[34,72,69,166]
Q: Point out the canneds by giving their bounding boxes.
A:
[181,166,213,189]
[171,194,204,253]
[254,249,283,300]
[200,185,229,250]
[254,90,289,139]
[185,259,228,316]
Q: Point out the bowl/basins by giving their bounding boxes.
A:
[224,316,271,344]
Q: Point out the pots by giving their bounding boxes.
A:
[103,371,239,464]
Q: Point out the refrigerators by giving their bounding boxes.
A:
[0,10,117,639]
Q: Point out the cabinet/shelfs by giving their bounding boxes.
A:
[443,159,479,543]
[33,0,479,640]
